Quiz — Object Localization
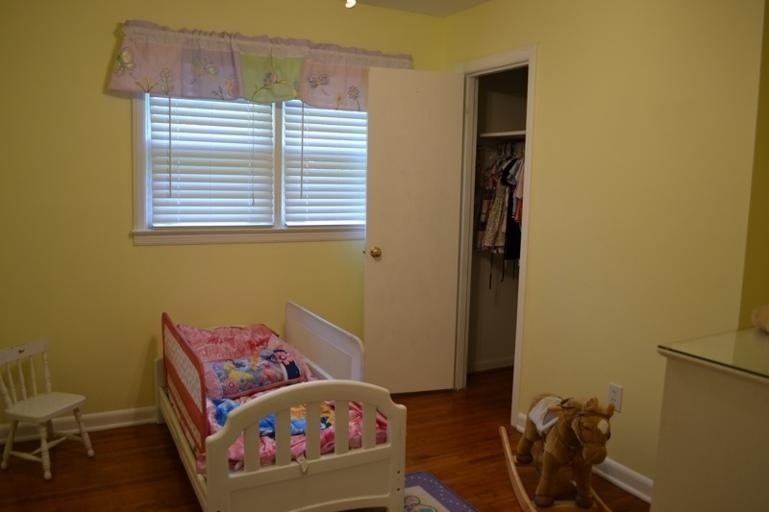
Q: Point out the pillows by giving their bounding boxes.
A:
[201,344,311,401]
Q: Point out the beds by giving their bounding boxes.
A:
[155,355,407,512]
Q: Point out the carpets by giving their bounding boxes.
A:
[406,471,480,512]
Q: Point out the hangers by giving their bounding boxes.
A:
[497,141,524,165]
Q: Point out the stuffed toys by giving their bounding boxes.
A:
[497,391,617,512]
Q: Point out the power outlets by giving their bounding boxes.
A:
[609,383,623,413]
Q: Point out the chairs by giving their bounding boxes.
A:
[0,340,95,481]
